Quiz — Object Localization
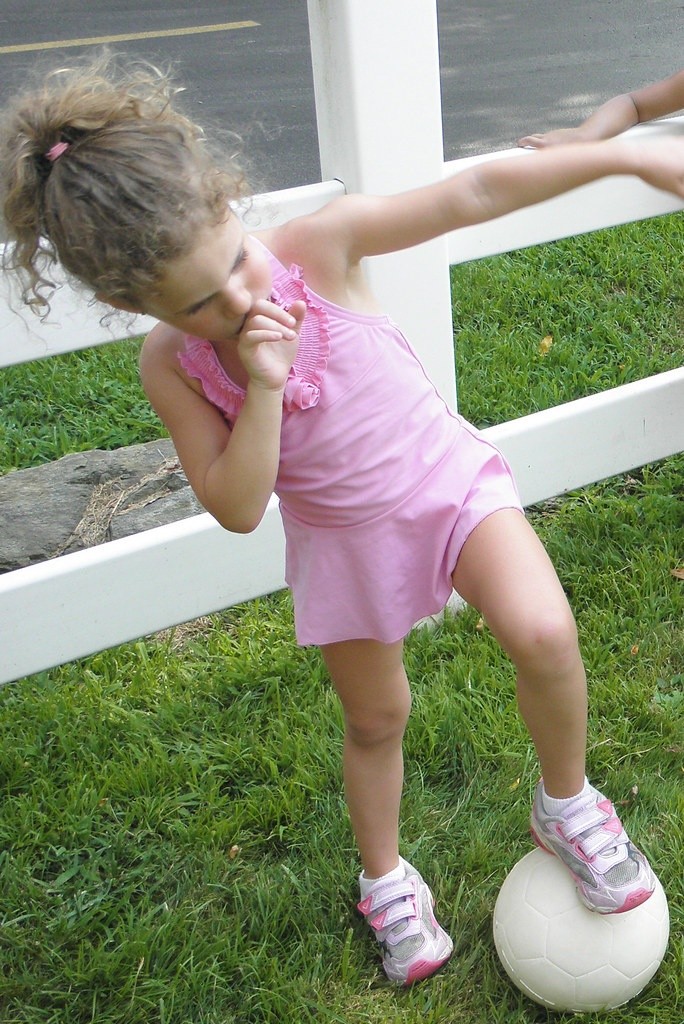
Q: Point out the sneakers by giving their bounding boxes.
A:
[358,856,455,986]
[530,779,656,915]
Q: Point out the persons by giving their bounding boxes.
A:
[0,40,684,986]
[518,66,684,149]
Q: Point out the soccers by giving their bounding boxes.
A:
[494,845,670,1013]
